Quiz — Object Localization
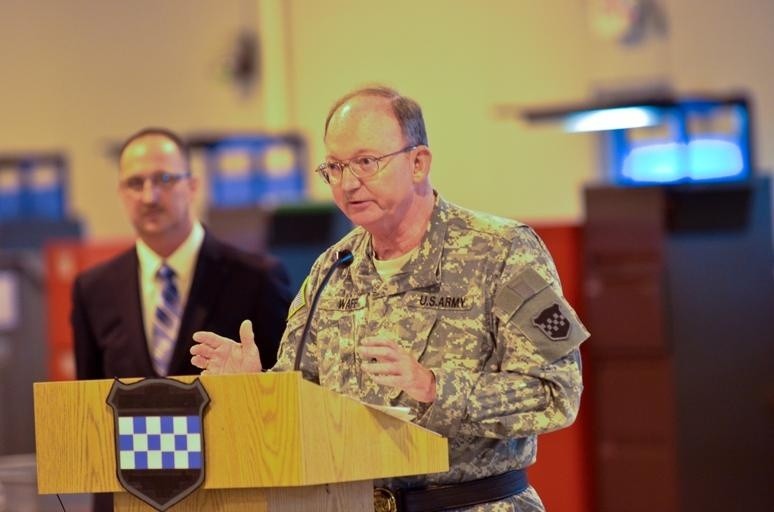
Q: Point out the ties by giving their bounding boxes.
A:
[150,265,182,379]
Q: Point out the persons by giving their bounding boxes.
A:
[68,128,294,512]
[188,85,593,512]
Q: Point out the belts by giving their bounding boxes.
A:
[372,469,528,512]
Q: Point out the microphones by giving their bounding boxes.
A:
[294,249,354,370]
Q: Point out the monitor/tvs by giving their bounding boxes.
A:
[600,95,751,193]
[204,133,306,209]
[0,152,66,223]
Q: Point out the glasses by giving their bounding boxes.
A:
[122,174,193,190]
[312,144,416,186]
